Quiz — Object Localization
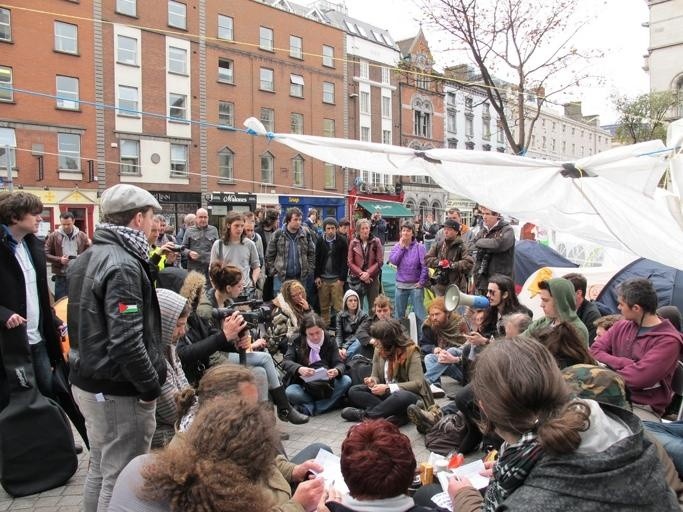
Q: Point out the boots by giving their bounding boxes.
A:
[266,382,309,426]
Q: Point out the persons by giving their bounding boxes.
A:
[256,208,277,254]
[338,218,352,243]
[427,220,474,294]
[243,212,254,222]
[594,313,622,342]
[533,322,593,369]
[456,277,591,416]
[431,207,471,247]
[371,214,386,245]
[447,362,682,512]
[422,213,440,252]
[155,214,181,270]
[474,335,680,512]
[325,418,433,511]
[65,182,167,512]
[244,221,265,300]
[342,318,435,427]
[285,312,352,417]
[183,208,218,275]
[269,279,312,356]
[421,301,484,398]
[0,189,91,455]
[462,273,532,389]
[176,212,196,268]
[254,208,265,219]
[304,209,318,232]
[407,309,528,435]
[371,205,382,227]
[176,363,324,512]
[357,294,394,357]
[209,212,262,296]
[591,275,683,422]
[197,261,309,440]
[110,400,276,512]
[315,218,347,329]
[266,205,315,304]
[563,274,601,342]
[335,289,368,363]
[148,287,195,452]
[157,265,251,389]
[45,210,90,300]
[347,218,383,316]
[471,209,481,227]
[392,222,426,346]
[472,204,516,296]
[146,217,181,288]
[419,296,469,397]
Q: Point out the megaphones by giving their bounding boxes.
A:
[444,284,489,313]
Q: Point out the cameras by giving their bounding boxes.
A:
[211,298,272,336]
[430,271,450,286]
[478,253,492,275]
[171,243,186,253]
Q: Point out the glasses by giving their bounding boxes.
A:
[484,289,503,295]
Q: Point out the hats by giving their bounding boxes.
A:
[439,220,461,232]
[338,289,362,312]
[155,286,188,343]
[279,280,301,307]
[545,278,579,324]
[157,267,205,316]
[100,184,163,214]
[561,363,627,410]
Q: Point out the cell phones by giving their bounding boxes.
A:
[302,470,318,483]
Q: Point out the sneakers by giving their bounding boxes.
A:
[407,404,445,433]
[341,406,364,421]
[413,400,427,435]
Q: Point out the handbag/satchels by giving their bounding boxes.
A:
[425,240,450,284]
[346,239,371,296]
